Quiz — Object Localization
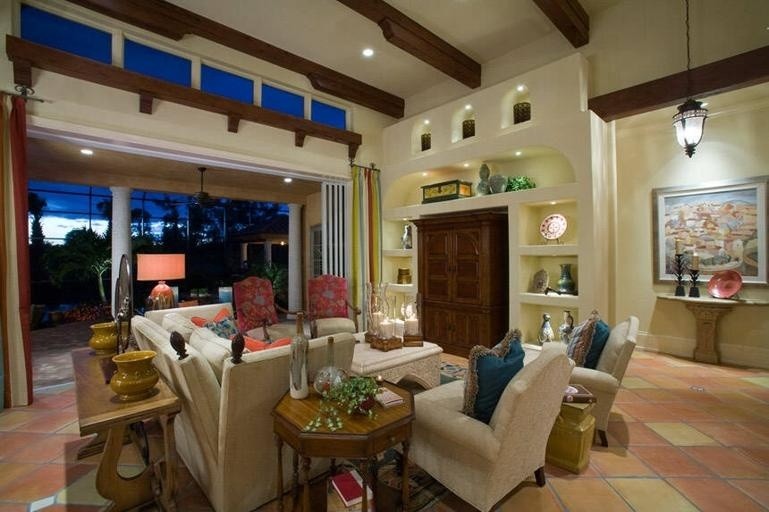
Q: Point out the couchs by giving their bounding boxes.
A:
[568,316,639,448]
[391,341,577,512]
[130,301,357,512]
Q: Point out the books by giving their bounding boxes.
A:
[332,469,373,508]
[373,386,404,407]
[563,384,597,403]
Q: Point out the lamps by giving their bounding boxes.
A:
[672,0,708,159]
[136,253,186,311]
[186,167,216,210]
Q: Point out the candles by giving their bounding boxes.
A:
[675,240,682,254]
[692,255,699,270]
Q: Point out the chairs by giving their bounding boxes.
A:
[232,276,305,343]
[305,274,361,339]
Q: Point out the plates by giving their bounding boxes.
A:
[539,213,568,239]
[532,269,549,292]
[706,270,744,298]
[399,296,418,321]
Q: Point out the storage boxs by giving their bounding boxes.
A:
[419,178,473,204]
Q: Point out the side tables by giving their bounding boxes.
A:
[545,401,596,475]
[271,375,416,512]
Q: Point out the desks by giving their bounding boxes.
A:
[70,344,183,512]
[656,292,769,366]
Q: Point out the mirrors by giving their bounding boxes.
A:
[116,254,132,350]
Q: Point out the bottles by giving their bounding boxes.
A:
[289,310,310,400]
[399,224,412,249]
[555,263,575,294]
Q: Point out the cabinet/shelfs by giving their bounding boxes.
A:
[408,211,509,360]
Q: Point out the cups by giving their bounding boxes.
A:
[370,315,418,340]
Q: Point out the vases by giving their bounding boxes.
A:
[30,304,46,330]
[110,350,158,401]
[87,321,118,354]
[48,311,64,327]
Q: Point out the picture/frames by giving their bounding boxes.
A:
[651,174,769,290]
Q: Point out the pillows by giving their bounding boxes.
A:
[161,307,292,386]
[458,327,525,425]
[565,309,611,370]
